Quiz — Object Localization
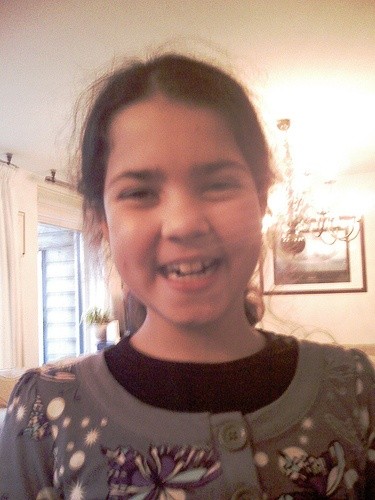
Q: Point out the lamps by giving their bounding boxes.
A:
[259,119,364,254]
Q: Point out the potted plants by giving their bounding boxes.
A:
[86,308,113,342]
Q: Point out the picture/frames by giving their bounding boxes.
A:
[258,215,368,294]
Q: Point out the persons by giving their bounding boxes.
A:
[1,35,374,500]
